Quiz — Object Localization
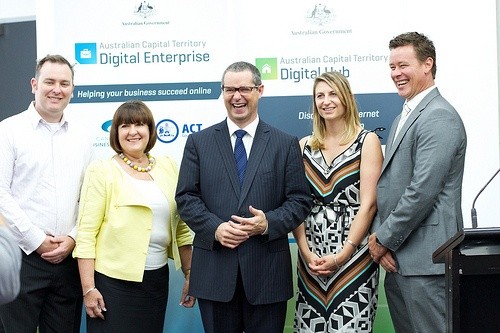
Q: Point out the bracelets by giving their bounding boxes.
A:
[346,238,357,248]
[375,238,382,246]
[83,286,96,296]
[183,269,191,275]
[334,256,339,268]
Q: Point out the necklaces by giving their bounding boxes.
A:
[121,153,155,172]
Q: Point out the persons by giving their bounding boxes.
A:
[72,101,196,333]
[292,71,384,333]
[0,54,93,333]
[368,32,468,333]
[175,62,313,333]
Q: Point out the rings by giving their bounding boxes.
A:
[371,255,374,258]
[241,220,246,224]
[94,308,98,311]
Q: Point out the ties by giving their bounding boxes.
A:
[234,129,248,190]
[392,105,411,143]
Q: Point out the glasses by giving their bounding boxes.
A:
[222,85,259,94]
